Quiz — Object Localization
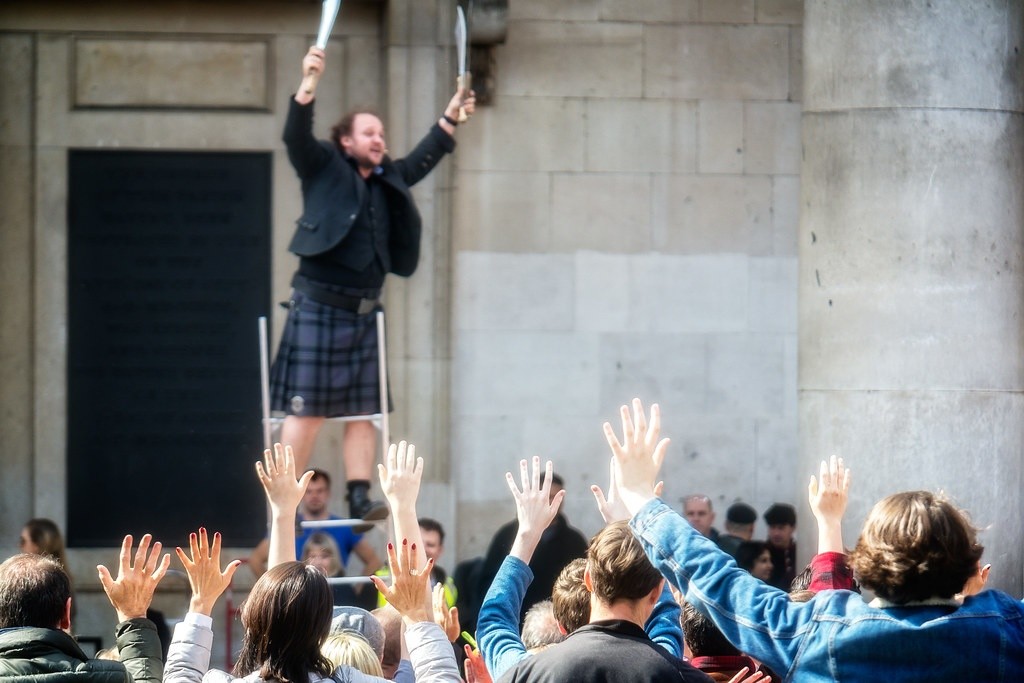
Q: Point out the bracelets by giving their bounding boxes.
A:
[443,111,460,127]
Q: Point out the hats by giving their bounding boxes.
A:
[727,503,757,524]
[763,503,797,526]
[330,606,386,664]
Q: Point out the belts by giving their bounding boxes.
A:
[288,273,381,315]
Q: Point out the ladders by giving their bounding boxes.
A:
[256,313,397,605]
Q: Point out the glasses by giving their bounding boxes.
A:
[687,496,709,504]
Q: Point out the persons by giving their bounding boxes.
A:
[0,398,1022,683]
[258,39,477,528]
[250,469,382,581]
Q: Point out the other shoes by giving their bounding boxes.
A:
[347,481,389,533]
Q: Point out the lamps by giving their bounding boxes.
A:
[461,0,512,105]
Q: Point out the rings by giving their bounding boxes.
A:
[409,569,418,575]
